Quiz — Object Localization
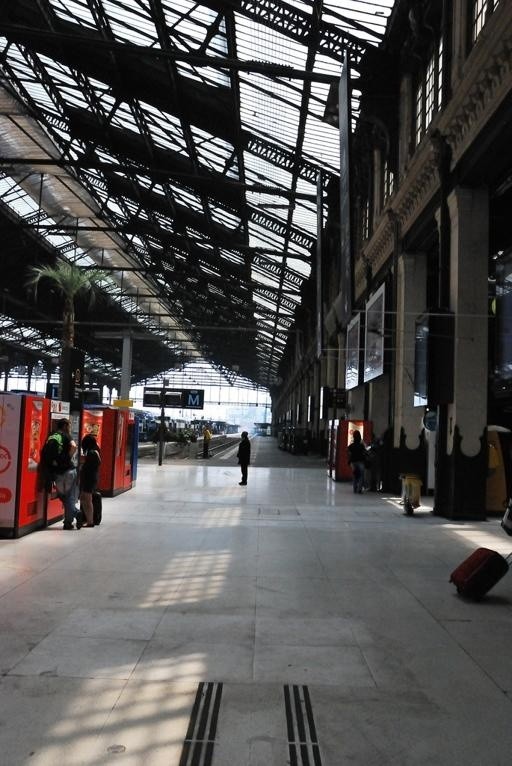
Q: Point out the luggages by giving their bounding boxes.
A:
[80,491,102,526]
[448,548,512,600]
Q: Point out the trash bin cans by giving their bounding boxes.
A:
[402,473,421,508]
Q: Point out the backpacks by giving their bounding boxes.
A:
[42,431,72,473]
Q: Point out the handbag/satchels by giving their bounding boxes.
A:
[501,497,512,537]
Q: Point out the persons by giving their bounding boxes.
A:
[78,435,102,528]
[44,418,85,530]
[203,424,212,460]
[347,431,366,494]
[237,431,251,485]
